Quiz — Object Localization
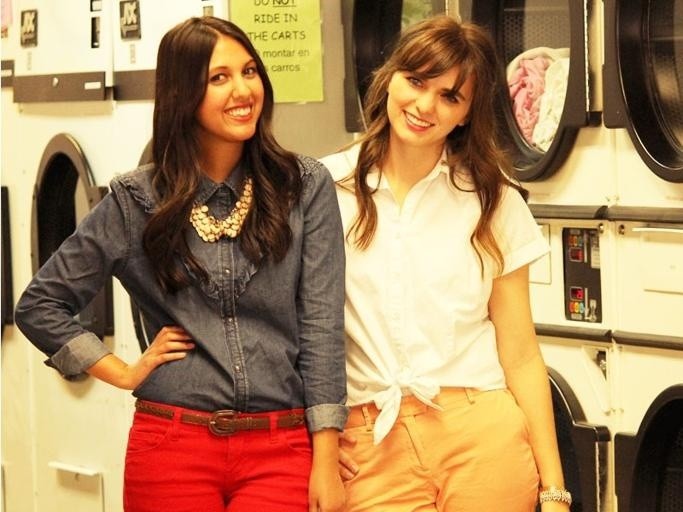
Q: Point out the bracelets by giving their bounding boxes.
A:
[538,488,573,507]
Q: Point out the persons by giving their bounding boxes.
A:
[12,16,349,512]
[314,16,573,512]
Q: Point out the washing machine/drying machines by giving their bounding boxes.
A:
[1,0,683,512]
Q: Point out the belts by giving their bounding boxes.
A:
[342,387,477,429]
[134,401,305,437]
